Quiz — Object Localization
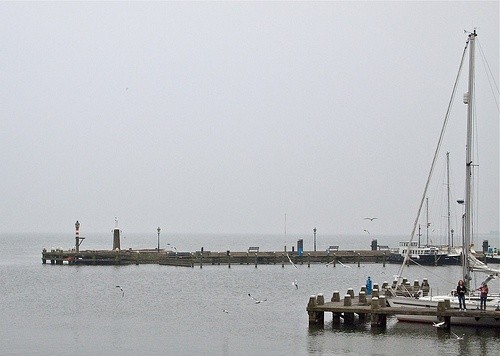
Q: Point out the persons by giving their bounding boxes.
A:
[457,280,467,312]
[366,277,372,295]
[479,283,488,310]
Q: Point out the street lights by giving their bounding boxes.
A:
[313,228,316,251]
[75,220,80,252]
[157,227,161,251]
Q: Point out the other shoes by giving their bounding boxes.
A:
[458,309,462,312]
[463,309,466,312]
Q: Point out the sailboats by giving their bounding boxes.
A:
[385,25,500,312]
[387,147,500,267]
